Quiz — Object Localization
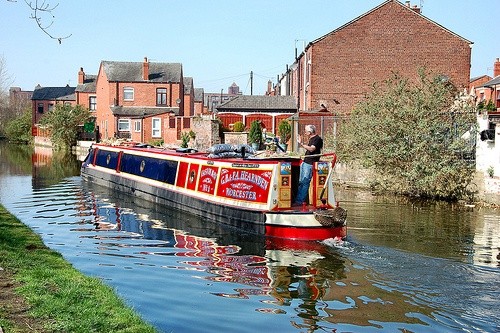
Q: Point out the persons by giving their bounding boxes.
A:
[291,124,323,207]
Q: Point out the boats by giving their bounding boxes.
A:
[79,132,348,242]
